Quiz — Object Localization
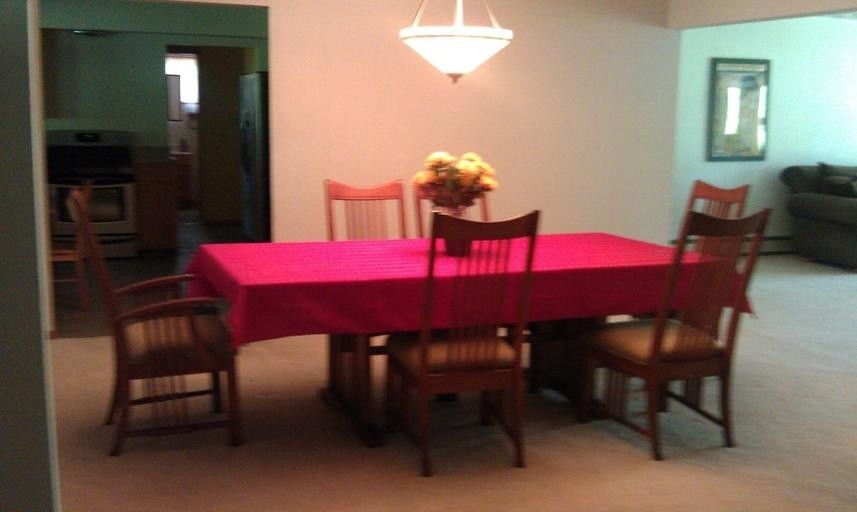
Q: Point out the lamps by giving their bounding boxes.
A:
[391,0,519,87]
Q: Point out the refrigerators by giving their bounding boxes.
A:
[235,71,270,239]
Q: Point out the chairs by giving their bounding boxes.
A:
[371,203,548,478]
[579,205,777,463]
[61,180,236,454]
[318,176,431,423]
[52,235,92,304]
[616,176,754,417]
[405,176,497,238]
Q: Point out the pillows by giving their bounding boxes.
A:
[821,176,855,196]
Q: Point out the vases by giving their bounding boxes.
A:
[439,215,473,254]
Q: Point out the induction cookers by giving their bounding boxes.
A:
[47,164,132,178]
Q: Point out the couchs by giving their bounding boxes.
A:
[778,159,855,268]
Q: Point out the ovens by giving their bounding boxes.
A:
[47,179,138,263]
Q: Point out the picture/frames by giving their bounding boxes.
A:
[707,53,772,160]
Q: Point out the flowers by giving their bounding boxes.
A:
[410,147,502,207]
